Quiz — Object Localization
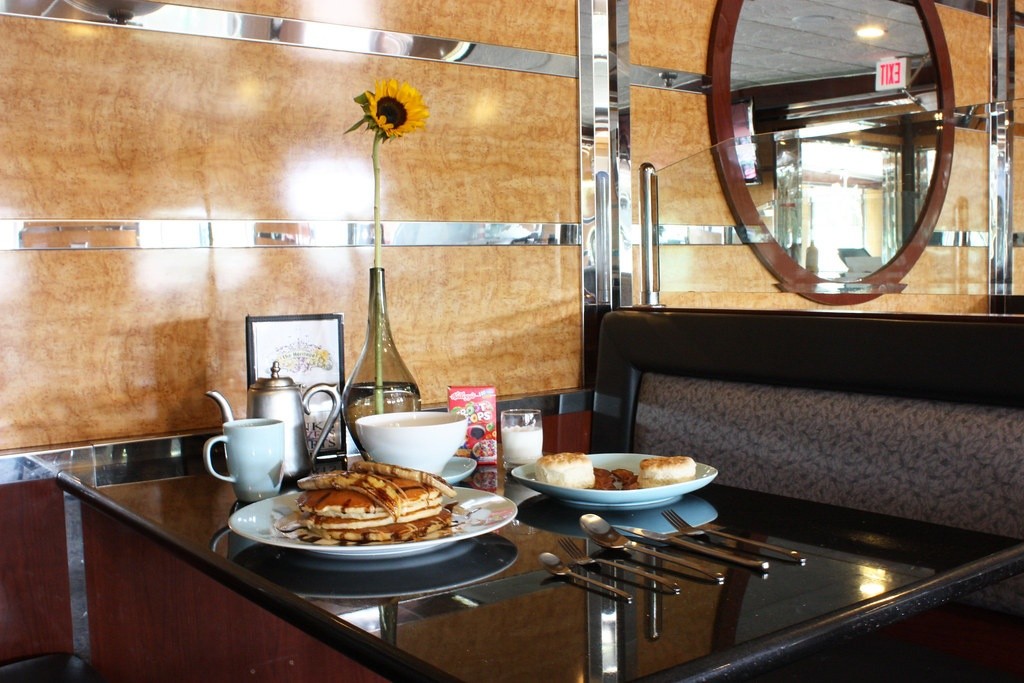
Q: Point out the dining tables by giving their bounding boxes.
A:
[57,441,1024,683]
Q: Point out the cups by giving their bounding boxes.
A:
[501,409,544,471]
[203,419,285,503]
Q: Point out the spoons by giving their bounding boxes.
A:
[537,552,634,600]
[579,514,726,581]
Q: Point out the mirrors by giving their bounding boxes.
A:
[706,0,956,306]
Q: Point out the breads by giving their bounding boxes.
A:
[637,455,697,488]
[534,451,595,489]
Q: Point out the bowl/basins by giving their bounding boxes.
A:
[355,412,468,475]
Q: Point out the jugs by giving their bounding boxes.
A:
[202,360,342,488]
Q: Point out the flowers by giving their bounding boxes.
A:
[342,77,429,415]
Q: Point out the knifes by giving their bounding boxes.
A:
[610,525,770,570]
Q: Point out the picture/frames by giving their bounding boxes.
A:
[244,312,348,457]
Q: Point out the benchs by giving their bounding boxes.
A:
[590,309,1024,683]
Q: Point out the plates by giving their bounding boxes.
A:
[441,457,477,485]
[510,453,718,511]
[228,486,517,556]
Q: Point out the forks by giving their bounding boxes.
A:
[557,535,681,591]
[660,507,807,562]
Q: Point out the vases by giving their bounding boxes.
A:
[341,269,422,461]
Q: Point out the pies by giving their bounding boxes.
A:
[295,474,452,541]
